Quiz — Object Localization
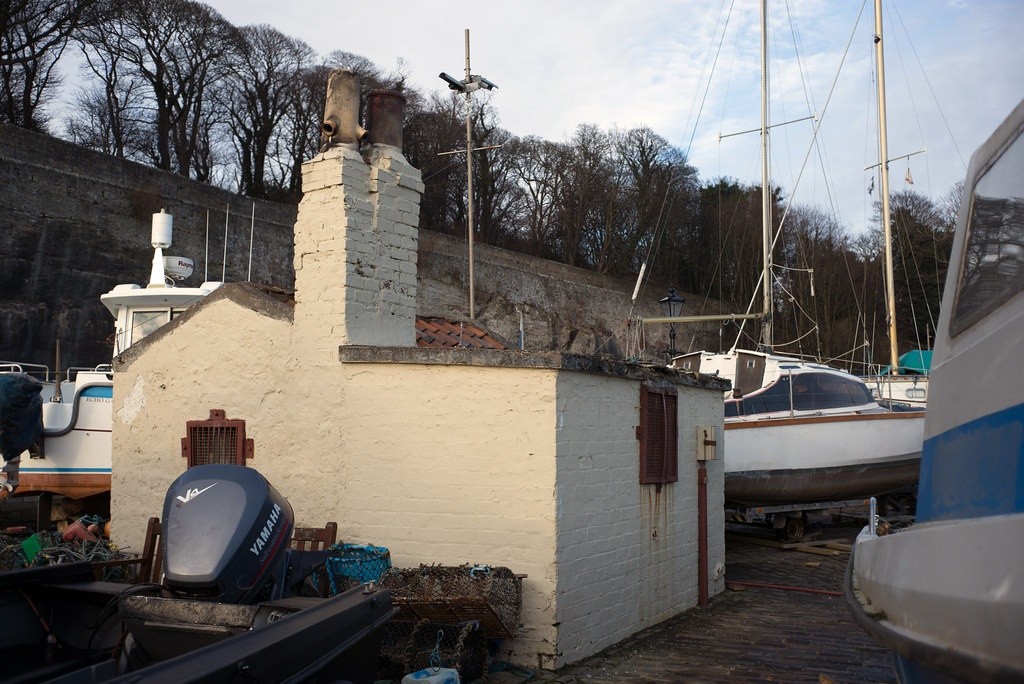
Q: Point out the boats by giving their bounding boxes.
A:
[841,96,1024,684]
[0,201,257,533]
[0,464,392,684]
[623,0,948,534]
